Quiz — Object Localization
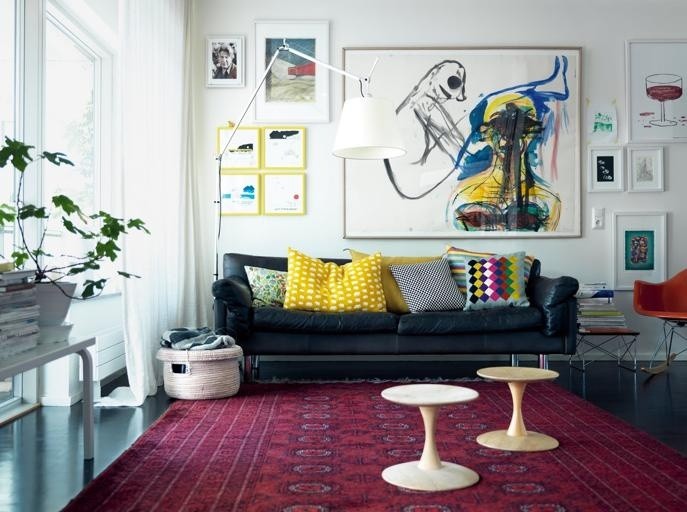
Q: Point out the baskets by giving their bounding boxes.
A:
[155,345,243,400]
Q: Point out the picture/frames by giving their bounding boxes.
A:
[255,18,331,124]
[339,46,582,240]
[612,212,669,291]
[216,121,261,170]
[216,172,261,216]
[262,126,307,170]
[586,144,624,192]
[206,34,246,90]
[626,146,664,193]
[261,172,307,216]
[625,39,686,144]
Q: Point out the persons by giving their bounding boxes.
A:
[213,41,237,80]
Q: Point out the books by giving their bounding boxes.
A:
[573,281,629,334]
[0,269,41,360]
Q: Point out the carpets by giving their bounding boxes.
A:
[57,379,687,511]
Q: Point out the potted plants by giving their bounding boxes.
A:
[0,137,151,325]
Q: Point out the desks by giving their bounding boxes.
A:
[0,336,99,465]
[379,384,480,493]
[476,366,561,453]
[569,329,639,375]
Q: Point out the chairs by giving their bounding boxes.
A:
[634,269,687,375]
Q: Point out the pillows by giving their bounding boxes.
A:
[389,258,466,313]
[444,245,534,301]
[244,265,287,307]
[345,248,442,313]
[463,251,530,311]
[283,247,387,313]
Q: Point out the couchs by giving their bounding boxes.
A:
[211,253,578,386]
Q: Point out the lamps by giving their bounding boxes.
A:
[212,38,406,306]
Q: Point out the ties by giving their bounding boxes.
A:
[224,70,229,77]
